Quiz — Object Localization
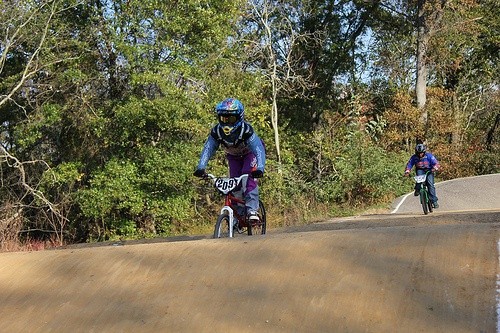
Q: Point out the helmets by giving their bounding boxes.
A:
[217,98,244,136]
[415,144,426,153]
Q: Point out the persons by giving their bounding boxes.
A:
[193,98,265,233]
[404,144,439,208]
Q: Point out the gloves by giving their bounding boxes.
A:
[251,170,263,179]
[193,168,205,178]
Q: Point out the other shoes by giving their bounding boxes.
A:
[414,190,419,196]
[433,202,438,208]
[248,212,259,221]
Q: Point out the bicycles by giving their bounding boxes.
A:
[193,171,267,239]
[403,169,438,214]
